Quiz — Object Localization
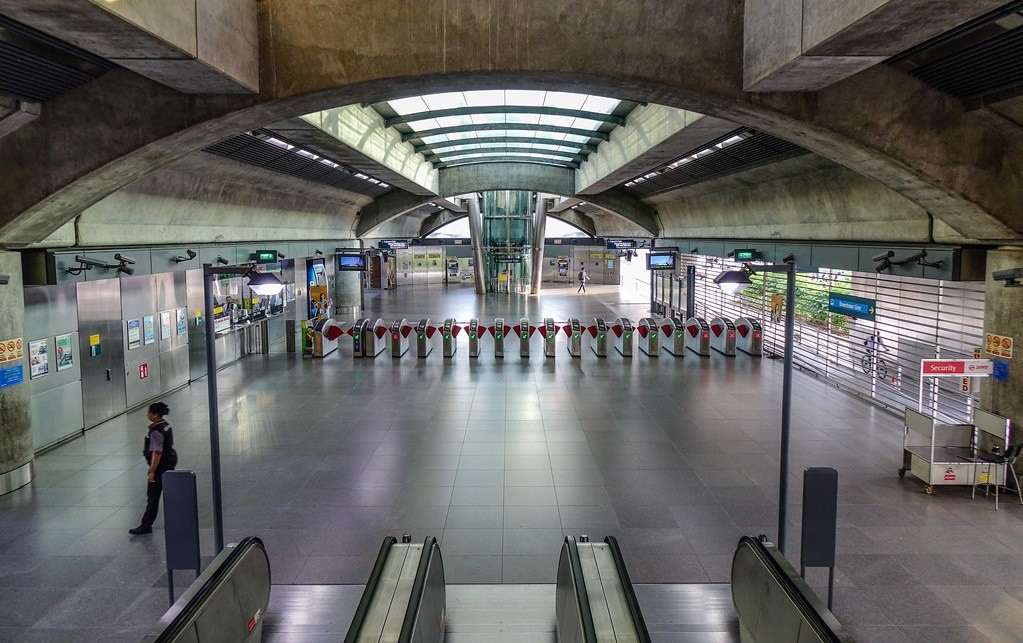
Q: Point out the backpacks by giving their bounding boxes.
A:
[578,271,584,280]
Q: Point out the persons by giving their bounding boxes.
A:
[576,267,590,294]
[222,296,239,316]
[317,293,329,314]
[388,270,394,290]
[128,401,178,535]
[864,331,889,367]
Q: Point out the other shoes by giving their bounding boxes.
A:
[584,291,587,294]
[577,292,580,294]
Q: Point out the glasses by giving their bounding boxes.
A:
[227,299,230,301]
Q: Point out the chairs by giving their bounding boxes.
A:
[972,443,1023,511]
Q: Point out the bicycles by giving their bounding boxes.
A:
[862,350,889,379]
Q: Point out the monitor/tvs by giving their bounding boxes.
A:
[646,253,681,270]
[338,254,366,271]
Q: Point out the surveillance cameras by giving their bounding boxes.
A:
[991,268,1023,280]
[114,254,136,264]
[0,274,10,284]
[873,251,895,262]
[75,256,107,267]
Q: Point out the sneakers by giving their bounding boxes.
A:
[129,525,152,534]
